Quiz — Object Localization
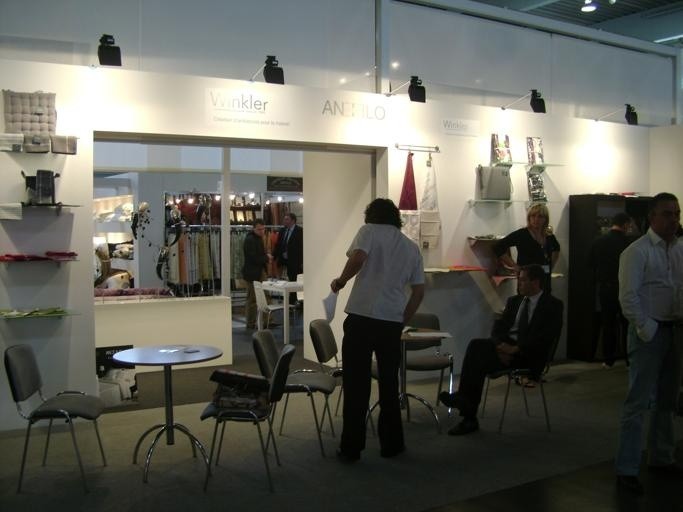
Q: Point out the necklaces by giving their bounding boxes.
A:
[530,227,545,249]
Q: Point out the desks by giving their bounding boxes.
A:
[361,327,452,437]
[109,344,221,485]
[262,282,304,347]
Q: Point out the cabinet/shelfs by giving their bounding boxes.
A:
[1,131,82,323]
[467,158,563,290]
[567,195,652,364]
[92,193,136,291]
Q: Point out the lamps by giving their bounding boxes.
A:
[594,101,640,133]
[245,57,288,89]
[388,75,426,104]
[91,31,122,70]
[499,87,548,117]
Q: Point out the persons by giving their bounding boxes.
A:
[237,217,277,336]
[436,261,564,436]
[490,199,562,388]
[327,196,426,465]
[271,211,303,324]
[590,209,642,374]
[616,188,683,498]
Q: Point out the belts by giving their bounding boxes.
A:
[657,319,682,328]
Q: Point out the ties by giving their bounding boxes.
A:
[283,227,290,248]
[517,296,530,350]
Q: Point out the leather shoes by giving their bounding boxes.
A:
[246,321,283,330]
[438,389,480,437]
[379,443,407,459]
[334,448,362,464]
[615,475,646,496]
[645,460,682,480]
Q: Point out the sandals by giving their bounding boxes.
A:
[514,376,537,388]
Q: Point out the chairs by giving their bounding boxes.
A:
[399,311,453,418]
[252,278,296,340]
[202,343,296,491]
[1,341,107,496]
[252,331,333,463]
[309,317,376,435]
[480,316,566,434]
